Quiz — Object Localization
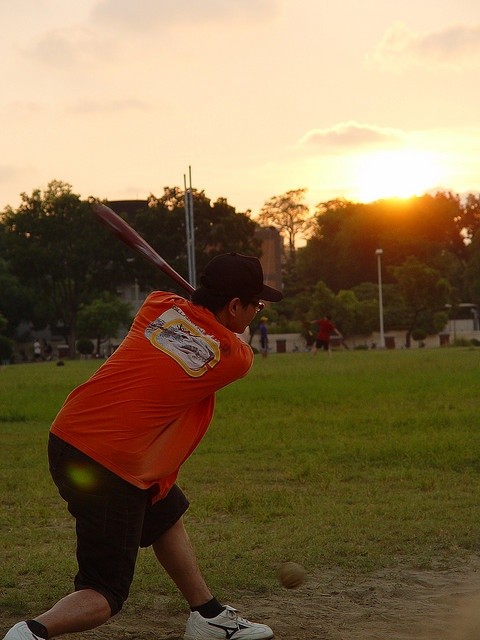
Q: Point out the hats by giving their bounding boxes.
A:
[199,252,283,302]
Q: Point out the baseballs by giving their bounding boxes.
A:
[280,562,305,587]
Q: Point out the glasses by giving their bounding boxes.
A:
[243,298,265,313]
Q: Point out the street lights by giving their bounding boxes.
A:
[376,248,386,349]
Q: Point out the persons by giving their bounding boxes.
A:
[259,317,270,358]
[42,338,53,360]
[33,338,42,361]
[0,252,274,639]
[308,314,344,357]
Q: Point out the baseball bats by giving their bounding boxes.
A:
[90,200,196,296]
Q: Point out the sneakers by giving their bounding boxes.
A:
[2,622,47,640]
[183,605,274,640]
